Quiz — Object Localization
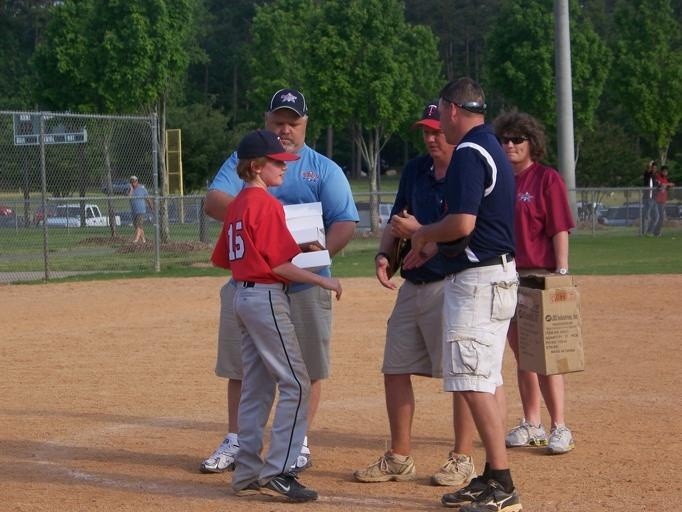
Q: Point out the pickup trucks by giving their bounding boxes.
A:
[40,203,121,229]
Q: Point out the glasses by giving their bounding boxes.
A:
[444,97,487,115]
[500,135,529,144]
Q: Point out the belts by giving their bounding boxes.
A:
[407,277,445,285]
[242,281,286,290]
[464,253,513,270]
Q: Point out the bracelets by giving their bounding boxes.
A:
[375,252,393,266]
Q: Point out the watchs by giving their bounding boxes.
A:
[555,267,568,274]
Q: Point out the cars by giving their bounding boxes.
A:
[101,179,146,195]
[119,198,208,224]
[573,198,682,225]
[33,206,58,224]
[338,158,388,177]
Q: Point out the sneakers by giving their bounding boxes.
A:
[430,450,477,487]
[441,473,487,506]
[505,418,549,446]
[199,436,317,499]
[459,480,523,512]
[549,425,575,453]
[354,449,415,482]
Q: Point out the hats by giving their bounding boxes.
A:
[270,88,307,117]
[238,131,300,161]
[413,102,442,130]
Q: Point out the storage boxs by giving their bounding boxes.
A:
[515,275,585,376]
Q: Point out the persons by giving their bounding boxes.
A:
[642,161,660,236]
[493,110,576,454]
[127,176,152,243]
[355,103,478,486]
[210,129,342,503]
[401,78,522,512]
[200,89,360,473]
[650,166,675,235]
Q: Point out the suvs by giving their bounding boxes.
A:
[354,200,395,230]
[0,203,15,219]
